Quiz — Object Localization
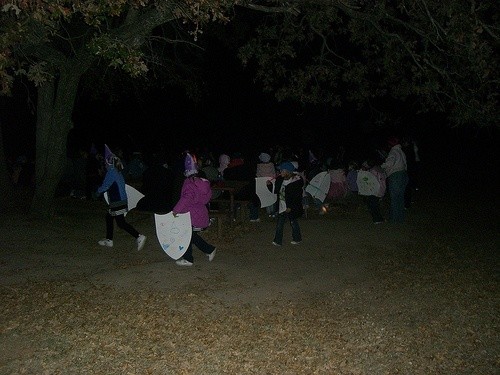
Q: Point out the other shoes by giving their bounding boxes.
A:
[176,259,193,267]
[208,248,216,262]
[271,241,281,248]
[137,234,146,250]
[291,240,303,244]
[98,239,113,247]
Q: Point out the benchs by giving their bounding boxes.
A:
[212,199,251,220]
[133,209,228,237]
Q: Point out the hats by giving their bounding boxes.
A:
[259,153,271,163]
[104,144,113,160]
[276,162,295,172]
[184,153,199,177]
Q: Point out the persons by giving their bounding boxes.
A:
[79,127,102,200]
[401,136,417,210]
[380,136,409,223]
[357,160,386,225]
[309,158,321,183]
[173,166,217,266]
[266,162,305,247]
[94,156,147,251]
[337,145,347,161]
[218,154,260,222]
[347,161,358,194]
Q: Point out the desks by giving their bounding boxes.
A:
[211,180,247,227]
